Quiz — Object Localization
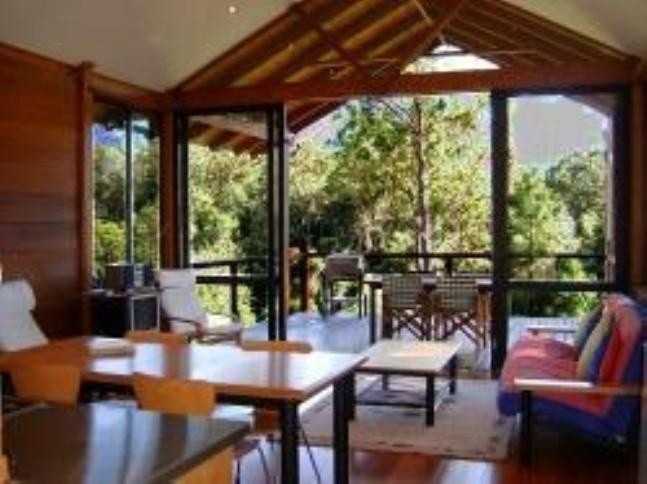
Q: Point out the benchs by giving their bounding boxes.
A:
[499,291,645,469]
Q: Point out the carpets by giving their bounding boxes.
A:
[266,372,518,462]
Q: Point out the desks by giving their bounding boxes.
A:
[2,338,324,481]
[0,334,370,484]
[362,272,502,345]
[1,394,249,483]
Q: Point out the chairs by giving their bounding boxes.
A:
[158,266,245,344]
[218,341,322,480]
[317,253,366,324]
[10,357,78,407]
[133,373,271,482]
[126,330,189,345]
[379,268,491,352]
